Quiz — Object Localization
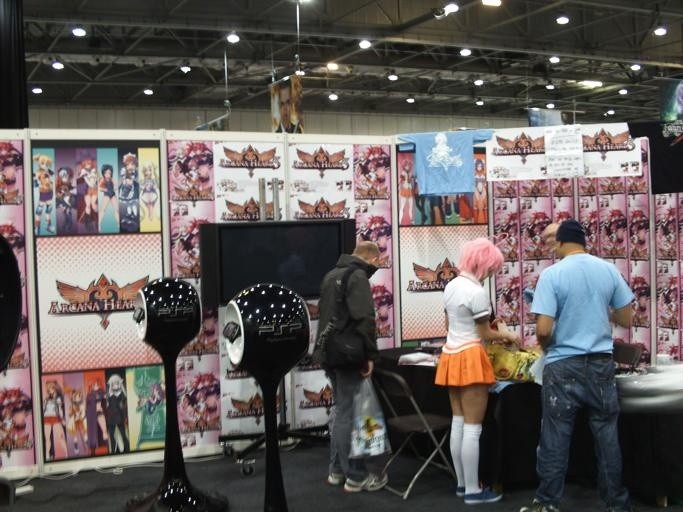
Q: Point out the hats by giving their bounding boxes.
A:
[556,220,585,246]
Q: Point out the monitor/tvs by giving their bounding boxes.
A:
[199,219,356,308]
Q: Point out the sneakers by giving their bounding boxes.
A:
[326,472,344,486]
[455,486,465,498]
[520,495,559,512]
[344,471,389,493]
[464,488,504,504]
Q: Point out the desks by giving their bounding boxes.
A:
[378,344,683,506]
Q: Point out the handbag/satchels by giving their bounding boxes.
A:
[315,315,365,370]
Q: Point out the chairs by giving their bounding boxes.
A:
[373,365,459,499]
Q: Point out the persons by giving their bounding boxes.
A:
[313,240,389,493]
[432,238,523,506]
[517,220,637,512]
[271,80,302,134]
